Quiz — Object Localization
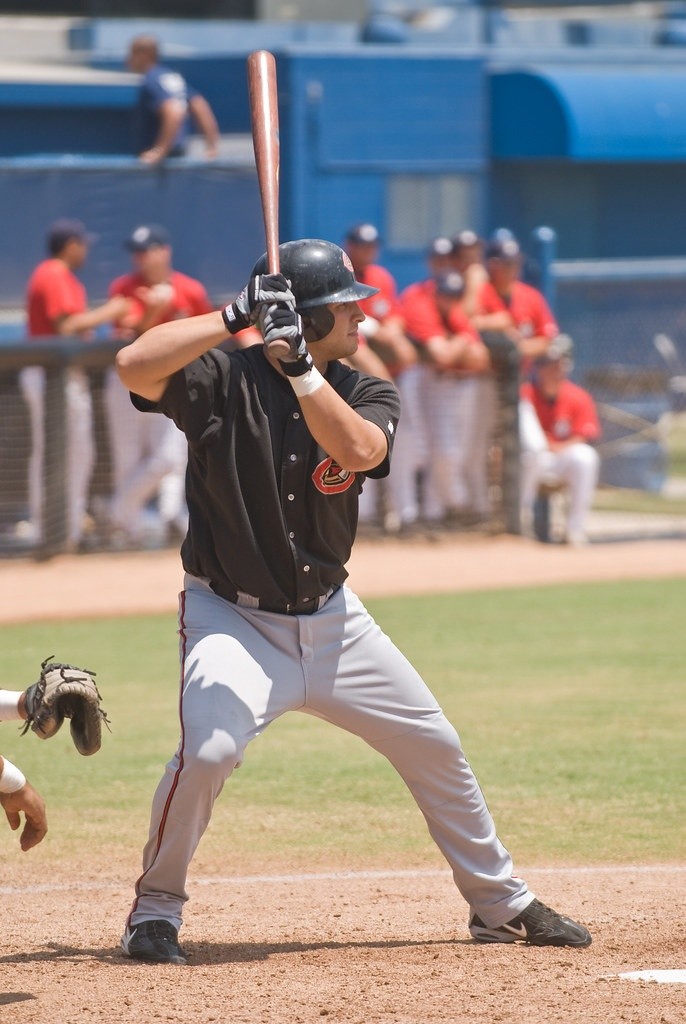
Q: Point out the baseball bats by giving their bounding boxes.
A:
[247,48,291,359]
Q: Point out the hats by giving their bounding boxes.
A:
[128,224,172,251]
[430,226,524,294]
[348,220,381,244]
[49,219,93,249]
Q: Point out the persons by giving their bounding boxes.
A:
[128,34,221,162]
[119,237,591,965]
[104,221,216,543]
[0,655,105,849]
[20,216,132,556]
[343,221,599,544]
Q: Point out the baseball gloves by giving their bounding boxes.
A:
[19,653,113,757]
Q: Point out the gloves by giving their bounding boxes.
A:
[260,300,314,378]
[222,272,296,335]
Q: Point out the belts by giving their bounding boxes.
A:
[208,578,339,616]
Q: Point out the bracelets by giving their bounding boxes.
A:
[288,366,326,398]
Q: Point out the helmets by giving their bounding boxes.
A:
[251,238,381,310]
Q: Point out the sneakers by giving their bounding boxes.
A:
[469,896,594,948]
[120,918,189,965]
